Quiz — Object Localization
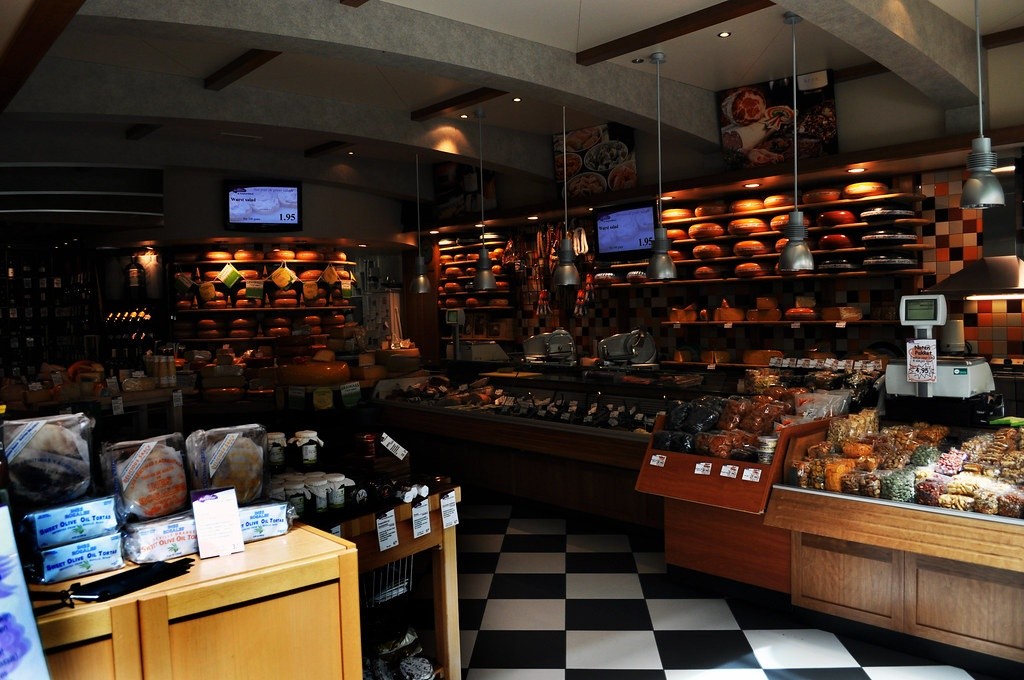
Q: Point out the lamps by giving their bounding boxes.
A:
[472,109,496,290]
[779,11,814,271]
[646,52,678,279]
[409,153,432,295]
[961,0,1005,210]
[553,105,579,286]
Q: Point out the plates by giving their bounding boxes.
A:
[555,127,637,200]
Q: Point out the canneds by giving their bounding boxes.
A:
[267,430,345,515]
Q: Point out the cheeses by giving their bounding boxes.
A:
[0,373,157,404]
[673,349,836,364]
[670,297,862,321]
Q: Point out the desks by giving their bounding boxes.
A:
[4,387,200,433]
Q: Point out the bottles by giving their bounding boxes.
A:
[105,310,177,388]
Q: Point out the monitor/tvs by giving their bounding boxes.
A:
[592,199,658,261]
[221,177,303,233]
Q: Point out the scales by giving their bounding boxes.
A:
[884,295,1006,430]
[446,307,513,361]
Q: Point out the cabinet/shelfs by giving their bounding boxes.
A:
[27,521,364,680]
[173,259,357,341]
[595,192,936,368]
[363,290,401,342]
[438,242,513,340]
